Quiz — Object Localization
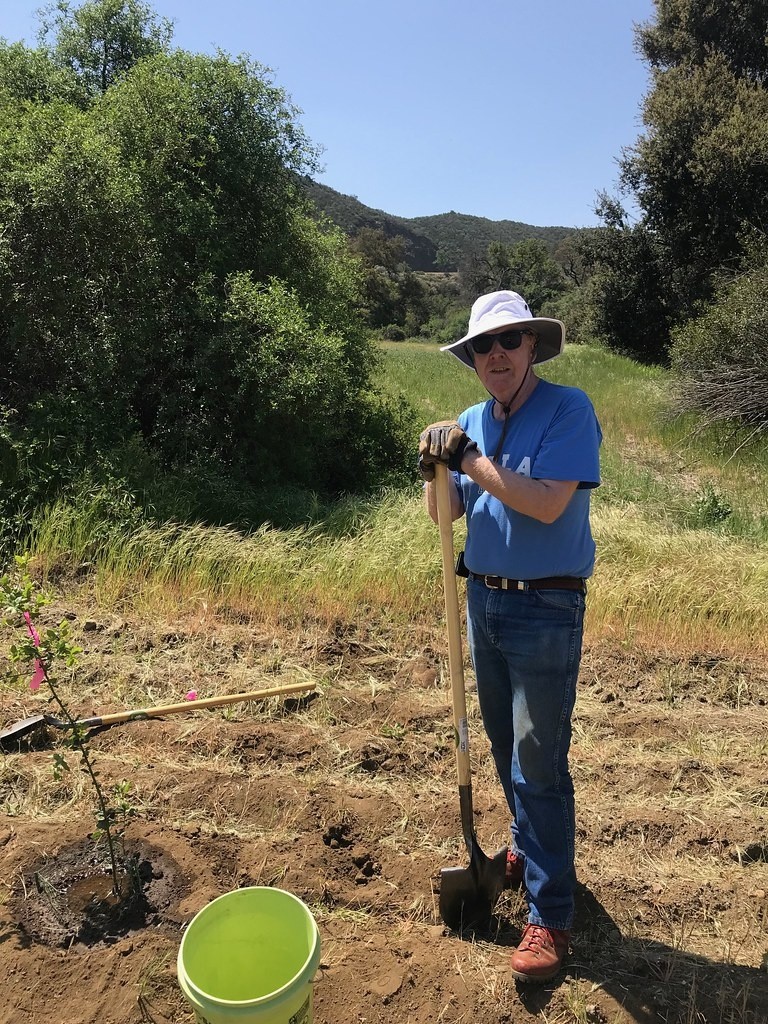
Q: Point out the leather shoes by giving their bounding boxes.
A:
[487,849,525,884]
[510,923,571,985]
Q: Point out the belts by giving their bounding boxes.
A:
[475,574,583,592]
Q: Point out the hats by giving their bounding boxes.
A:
[440,290,565,371]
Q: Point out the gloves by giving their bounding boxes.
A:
[418,420,482,482]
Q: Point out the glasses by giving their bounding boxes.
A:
[470,329,534,354]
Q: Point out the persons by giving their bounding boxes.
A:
[418,290,603,984]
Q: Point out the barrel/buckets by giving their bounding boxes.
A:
[176,886,325,1024]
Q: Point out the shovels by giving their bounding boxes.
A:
[436,462,511,931]
[1,679,319,744]
